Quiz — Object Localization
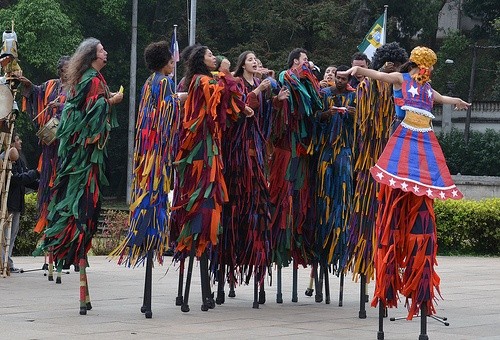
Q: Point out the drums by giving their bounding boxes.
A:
[36,116,60,146]
[0,84,14,121]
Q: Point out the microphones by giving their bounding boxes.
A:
[96,57,106,63]
[262,72,268,97]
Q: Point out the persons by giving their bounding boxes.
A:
[129,41,187,252]
[354,41,409,266]
[54,38,123,253]
[334,46,472,300]
[177,48,374,255]
[18,54,72,243]
[0,61,38,274]
